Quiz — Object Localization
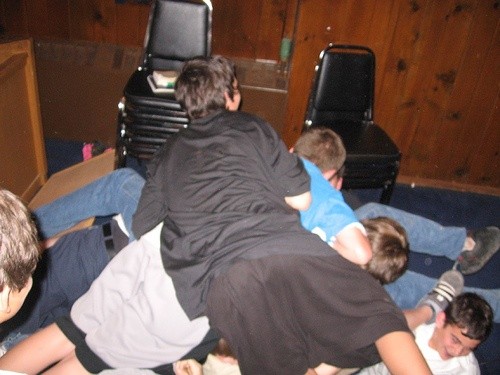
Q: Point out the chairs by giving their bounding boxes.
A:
[116,0,213,170]
[301,44,403,206]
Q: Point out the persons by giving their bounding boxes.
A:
[131,56,433,375]
[0,126,500,375]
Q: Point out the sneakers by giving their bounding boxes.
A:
[458,224,499,273]
[419,268,464,325]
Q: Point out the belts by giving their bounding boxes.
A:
[102,221,117,260]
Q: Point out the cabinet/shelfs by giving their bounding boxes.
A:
[0,36,47,204]
